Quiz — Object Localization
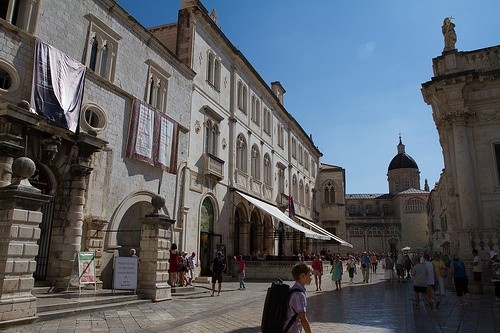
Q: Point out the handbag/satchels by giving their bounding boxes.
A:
[348,265,350,270]
[440,270,446,277]
[362,264,366,268]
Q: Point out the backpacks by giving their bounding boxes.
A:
[262,278,303,333]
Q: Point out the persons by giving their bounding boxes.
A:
[247,248,267,261]
[177,252,196,286]
[394,250,415,283]
[346,256,355,284]
[293,248,335,262]
[487,251,500,301]
[383,252,393,287]
[234,255,246,290]
[283,263,312,333]
[453,256,473,305]
[311,253,323,291]
[470,249,484,294]
[169,243,178,286]
[332,254,343,291]
[81,264,94,281]
[414,252,446,310]
[211,252,226,297]
[371,254,376,273]
[361,252,371,282]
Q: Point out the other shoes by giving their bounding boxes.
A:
[416,304,420,309]
[218,292,221,296]
[211,293,215,296]
[429,301,433,311]
[436,301,440,308]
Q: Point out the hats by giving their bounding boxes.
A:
[490,251,497,259]
[424,255,429,260]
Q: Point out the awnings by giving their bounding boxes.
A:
[293,213,354,248]
[230,187,333,240]
